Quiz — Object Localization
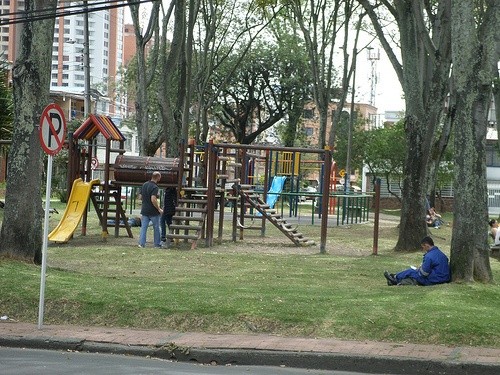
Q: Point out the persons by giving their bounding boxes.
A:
[138,171,163,248]
[384,237,451,286]
[489,219,500,246]
[160,187,177,243]
[426,207,449,229]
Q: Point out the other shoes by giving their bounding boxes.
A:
[384,270,397,285]
[435,226,441,229]
[138,242,145,248]
[154,245,161,249]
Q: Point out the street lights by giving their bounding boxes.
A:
[339,45,374,188]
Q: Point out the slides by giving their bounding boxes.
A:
[256,176,287,216]
[48,178,100,242]
